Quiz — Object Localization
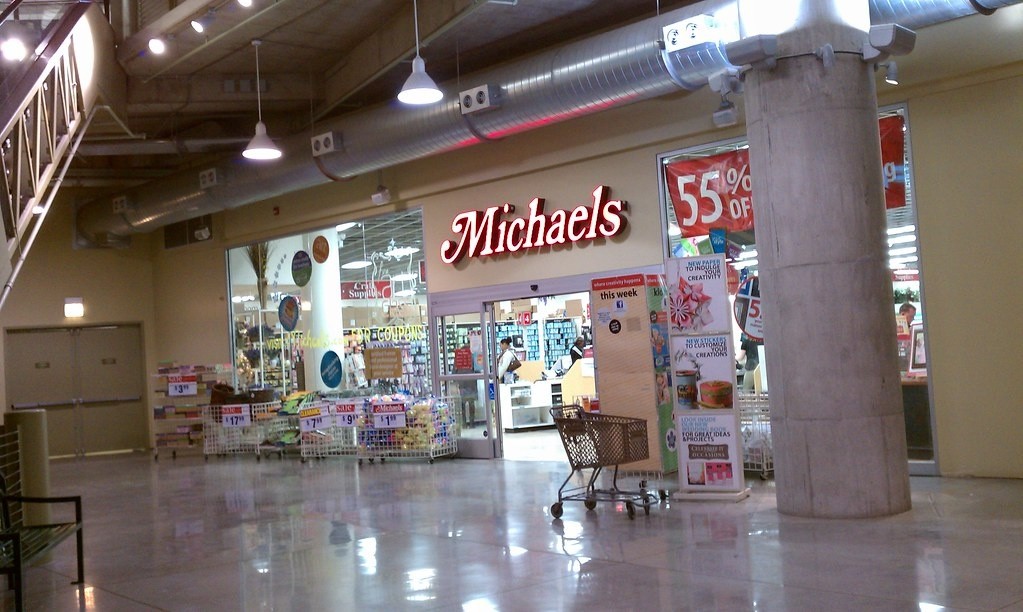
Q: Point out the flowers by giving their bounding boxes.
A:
[243,348,260,360]
[248,324,274,336]
[264,348,281,359]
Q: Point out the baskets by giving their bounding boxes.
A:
[209,379,275,424]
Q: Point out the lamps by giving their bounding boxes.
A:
[713,95,739,128]
[371,169,391,206]
[724,34,777,72]
[708,67,744,96]
[880,61,899,85]
[242,40,281,159]
[195,216,210,240]
[397,0,443,104]
[863,23,917,64]
[191,7,216,33]
[816,43,835,67]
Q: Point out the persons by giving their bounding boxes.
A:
[570,337,584,364]
[453,346,478,429]
[498,336,516,383]
[735,335,764,395]
[899,303,916,327]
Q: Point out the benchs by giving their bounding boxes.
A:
[0,424,84,612]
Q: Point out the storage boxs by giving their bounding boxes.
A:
[458,299,583,370]
[151,360,232,462]
[262,297,537,328]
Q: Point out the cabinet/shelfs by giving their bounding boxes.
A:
[233,316,581,394]
[499,380,563,433]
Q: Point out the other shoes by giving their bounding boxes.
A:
[469,423,476,428]
[462,424,467,429]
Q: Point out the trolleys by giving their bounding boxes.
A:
[736,386,776,483]
[548,405,658,522]
[297,401,357,464]
[200,401,286,463]
[147,370,237,463]
[355,397,456,468]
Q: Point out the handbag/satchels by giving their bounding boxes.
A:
[507,350,522,372]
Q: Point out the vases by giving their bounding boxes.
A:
[269,359,277,370]
[251,335,259,342]
[248,358,258,368]
